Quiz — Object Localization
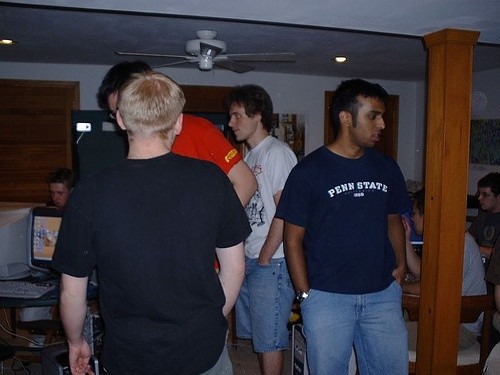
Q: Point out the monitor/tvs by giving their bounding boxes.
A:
[0,207,51,281]
[28,206,62,273]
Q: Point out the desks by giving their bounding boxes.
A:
[0,276,98,338]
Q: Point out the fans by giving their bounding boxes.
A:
[115,30,298,74]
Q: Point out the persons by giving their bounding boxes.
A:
[227,85,297,375]
[275,77,410,375]
[402,170,500,375]
[48,167,79,208]
[95,58,258,272]
[48,72,253,375]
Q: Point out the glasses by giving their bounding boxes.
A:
[110,107,119,120]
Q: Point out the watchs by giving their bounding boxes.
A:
[296,290,309,302]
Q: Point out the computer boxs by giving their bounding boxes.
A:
[292,323,358,375]
[40,344,99,375]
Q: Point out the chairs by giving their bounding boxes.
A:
[402,291,495,375]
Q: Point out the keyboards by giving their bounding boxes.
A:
[0,281,55,299]
